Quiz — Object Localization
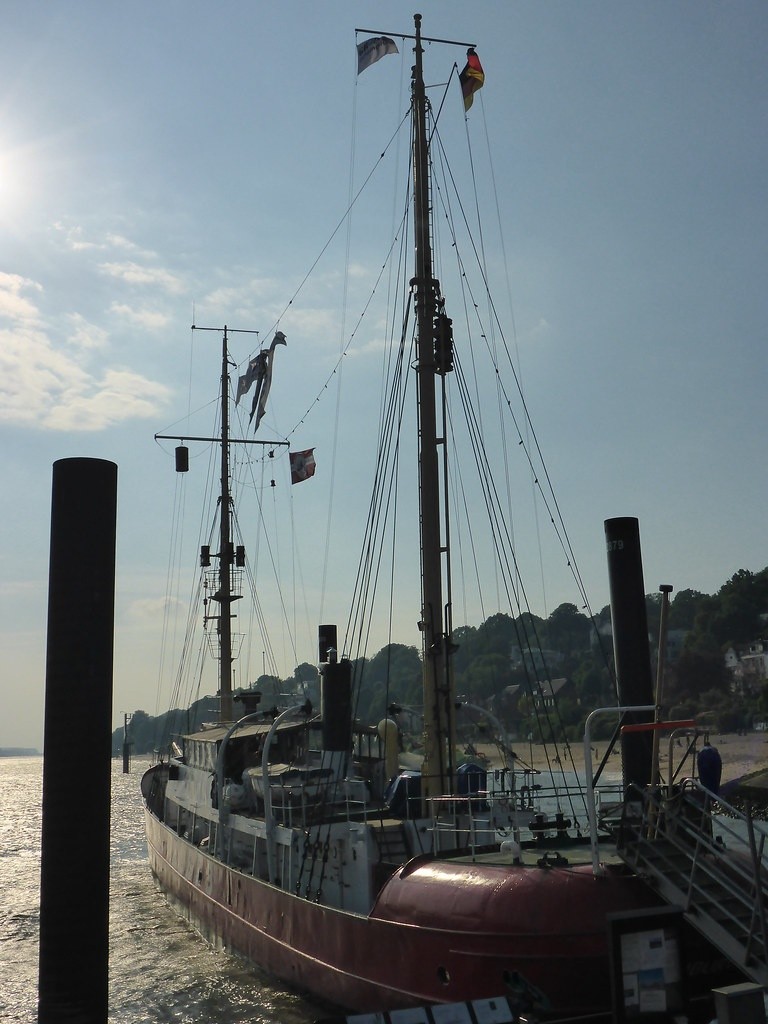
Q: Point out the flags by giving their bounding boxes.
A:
[460,48,485,113]
[289,446,317,485]
[355,36,400,75]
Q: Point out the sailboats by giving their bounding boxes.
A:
[141,13,726,1015]
